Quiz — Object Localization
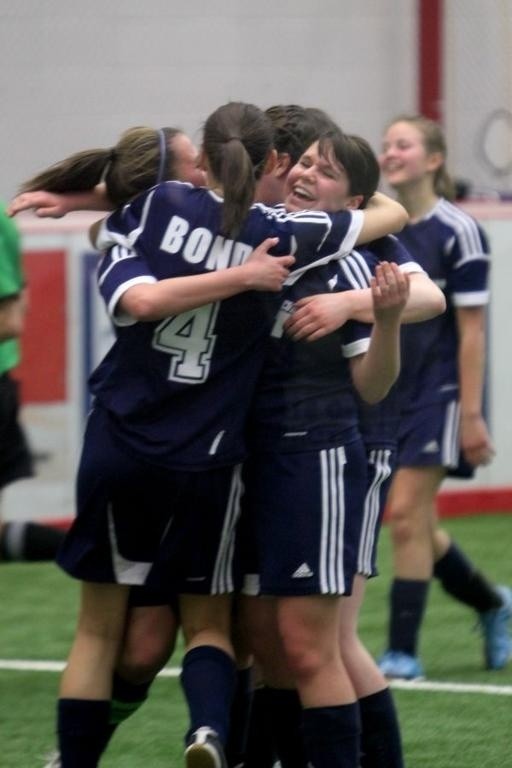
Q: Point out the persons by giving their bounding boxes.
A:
[12,100,447,768]
[379,113,512,679]
[0,202,35,488]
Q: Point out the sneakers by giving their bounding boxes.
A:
[184,725,225,768]
[379,649,425,681]
[479,586,512,670]
